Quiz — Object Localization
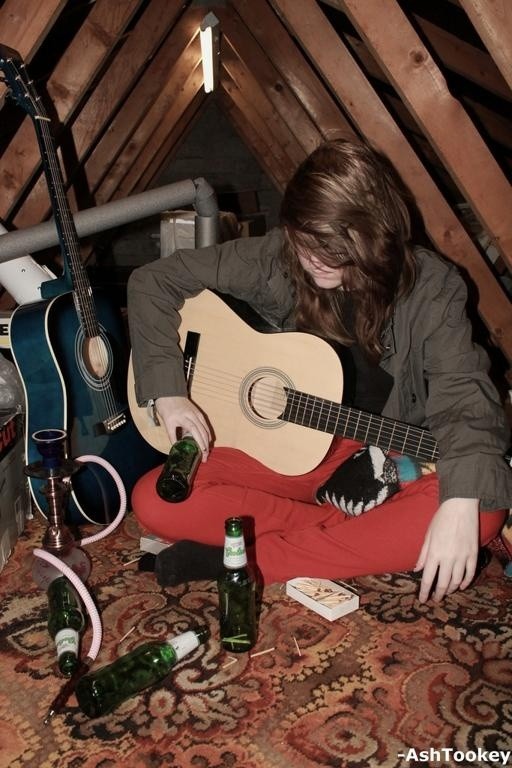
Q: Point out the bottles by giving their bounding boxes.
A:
[47,574,88,678]
[75,625,212,719]
[155,430,202,505]
[217,516,259,654]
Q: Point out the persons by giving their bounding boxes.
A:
[123,134,511,605]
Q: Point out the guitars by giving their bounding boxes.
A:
[127,288,441,476]
[0,44,170,526]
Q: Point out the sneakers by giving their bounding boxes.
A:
[413,546,495,598]
[156,538,225,589]
